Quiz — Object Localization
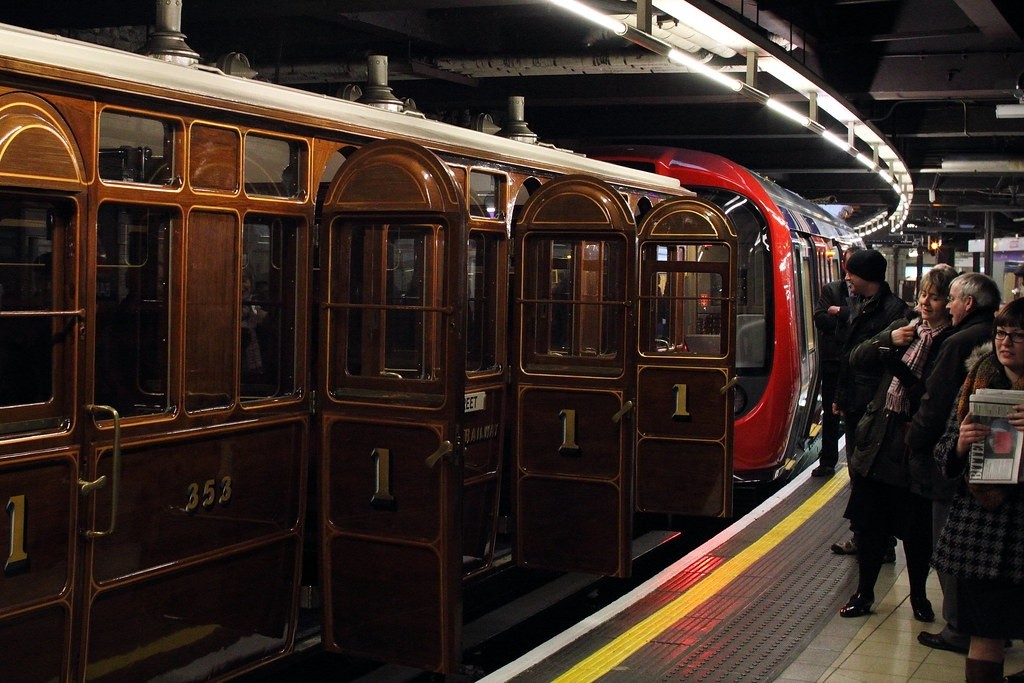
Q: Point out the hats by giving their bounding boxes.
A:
[846,250,887,282]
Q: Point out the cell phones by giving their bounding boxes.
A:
[879,347,890,354]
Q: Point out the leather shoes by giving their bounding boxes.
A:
[917,630,970,654]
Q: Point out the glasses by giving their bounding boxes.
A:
[946,295,972,303]
[992,330,1024,344]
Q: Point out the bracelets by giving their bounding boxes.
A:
[837,307,840,312]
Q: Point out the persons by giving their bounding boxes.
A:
[831,248,911,565]
[238,250,676,394]
[927,297,1024,683]
[840,264,960,621]
[811,246,869,477]
[900,273,1005,657]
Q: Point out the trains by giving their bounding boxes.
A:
[568,139,866,493]
[0,0,747,681]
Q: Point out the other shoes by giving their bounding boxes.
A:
[854,548,896,564]
[811,466,835,476]
[831,538,860,554]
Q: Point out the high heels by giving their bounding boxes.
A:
[910,597,935,623]
[840,592,875,618]
